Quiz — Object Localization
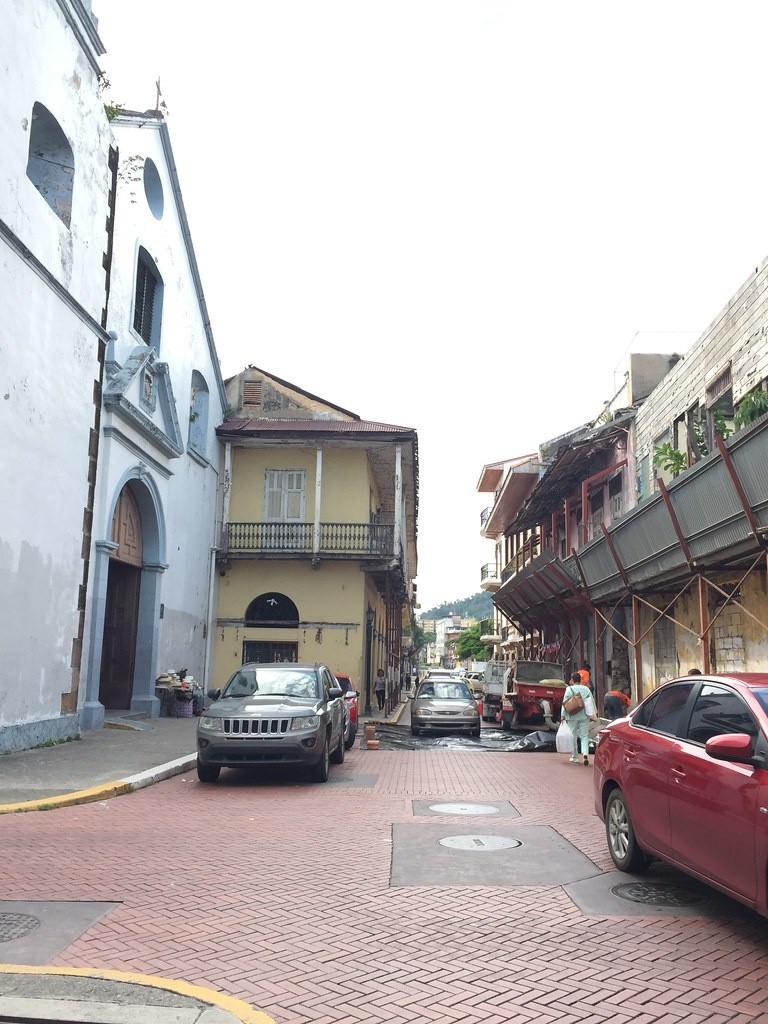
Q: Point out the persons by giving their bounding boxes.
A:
[373,669,385,712]
[560,672,597,765]
[578,664,594,694]
[605,691,630,719]
[415,674,419,687]
[181,668,203,715]
[413,667,416,675]
[406,674,411,691]
[688,668,701,675]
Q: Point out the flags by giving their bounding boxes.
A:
[540,639,564,653]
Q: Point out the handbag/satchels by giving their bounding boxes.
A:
[555,719,574,754]
[562,687,584,715]
[583,694,598,723]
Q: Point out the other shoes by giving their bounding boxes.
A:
[569,757,579,762]
[583,754,589,766]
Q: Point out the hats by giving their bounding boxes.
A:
[157,673,173,682]
[167,670,177,675]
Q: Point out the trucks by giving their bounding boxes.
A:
[482,659,513,721]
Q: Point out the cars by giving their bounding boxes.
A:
[334,674,359,734]
[428,671,450,679]
[451,671,484,694]
[593,673,768,918]
[407,679,480,737]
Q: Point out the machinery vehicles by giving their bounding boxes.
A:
[496,660,566,731]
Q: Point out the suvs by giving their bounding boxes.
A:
[196,663,348,782]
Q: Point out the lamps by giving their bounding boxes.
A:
[367,607,374,624]
